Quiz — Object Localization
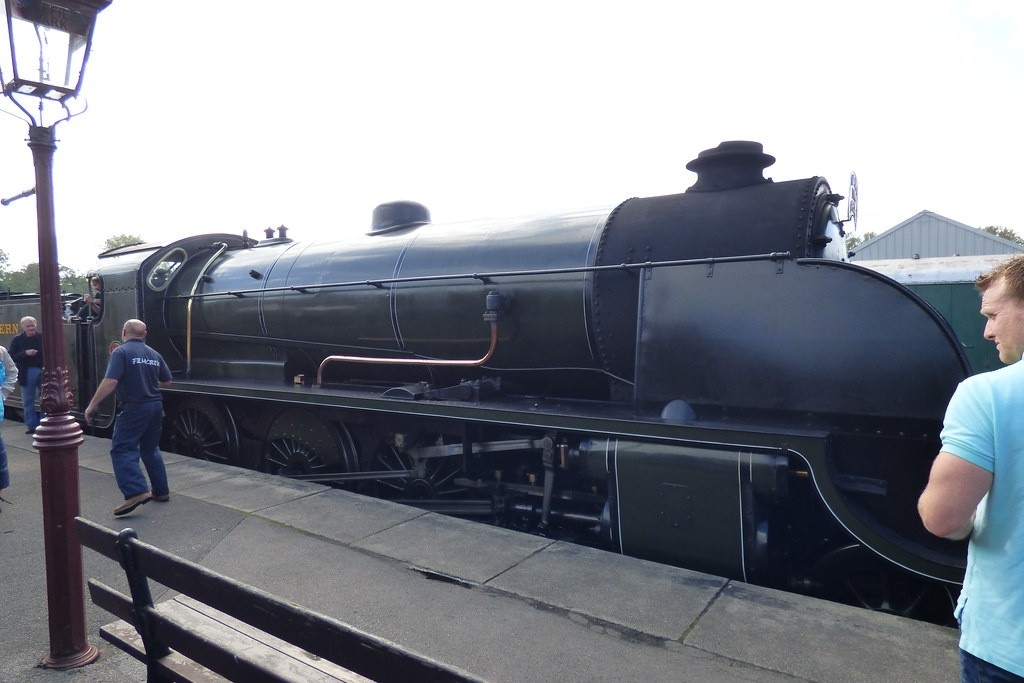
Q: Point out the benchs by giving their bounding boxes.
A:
[75,516,487,683]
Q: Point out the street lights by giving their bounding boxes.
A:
[0,0,115,673]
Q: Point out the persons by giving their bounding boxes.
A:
[82,318,174,516]
[8,316,42,433]
[0,346,19,490]
[916,257,1024,683]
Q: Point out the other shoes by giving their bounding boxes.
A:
[114,492,152,515]
[24,428,34,434]
[148,491,170,501]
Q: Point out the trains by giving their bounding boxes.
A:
[2,142,1024,592]
